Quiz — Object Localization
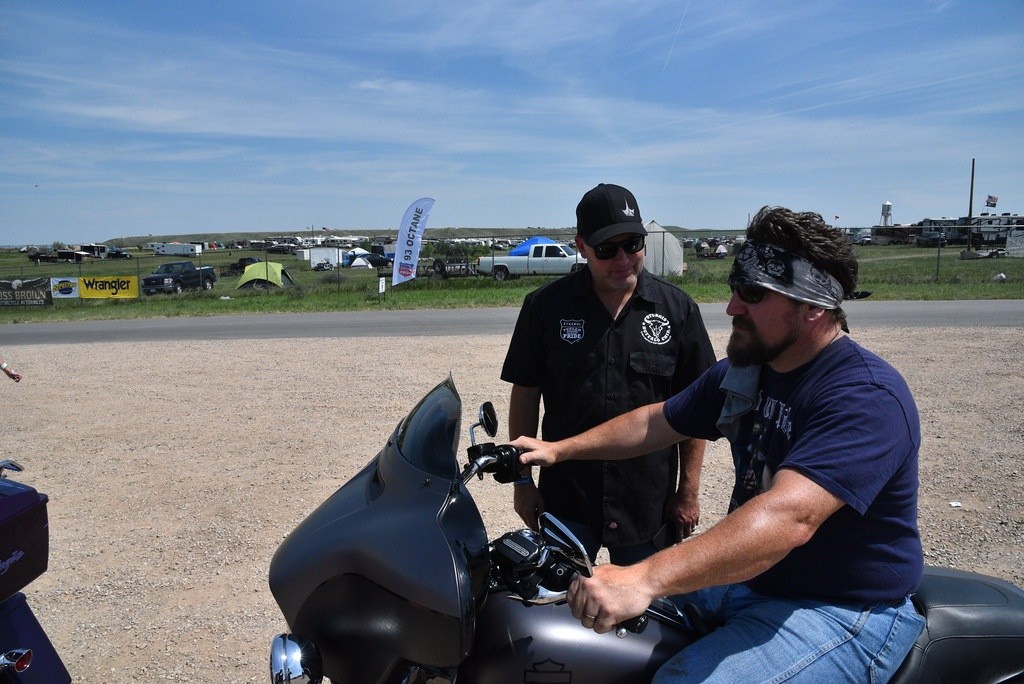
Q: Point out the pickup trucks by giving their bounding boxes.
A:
[476,243,588,282]
[916,232,948,248]
[28,252,50,263]
[141,261,217,296]
[229,256,263,272]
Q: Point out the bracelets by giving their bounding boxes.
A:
[1,363,8,370]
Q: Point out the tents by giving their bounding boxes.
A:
[639,219,685,276]
[235,262,296,291]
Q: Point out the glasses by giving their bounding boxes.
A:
[581,233,645,260]
[729,281,776,303]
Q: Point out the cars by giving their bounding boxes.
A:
[106,250,134,259]
[345,252,394,268]
[20,245,40,252]
[861,234,872,245]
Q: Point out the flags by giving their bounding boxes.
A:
[985,202,997,207]
[986,195,999,203]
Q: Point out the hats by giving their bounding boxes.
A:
[575,183,648,246]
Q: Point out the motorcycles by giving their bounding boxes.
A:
[266,371,1024,684]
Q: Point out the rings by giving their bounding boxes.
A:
[582,612,597,622]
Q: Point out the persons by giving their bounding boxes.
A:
[0,358,22,383]
[506,206,924,684]
[502,182,718,566]
